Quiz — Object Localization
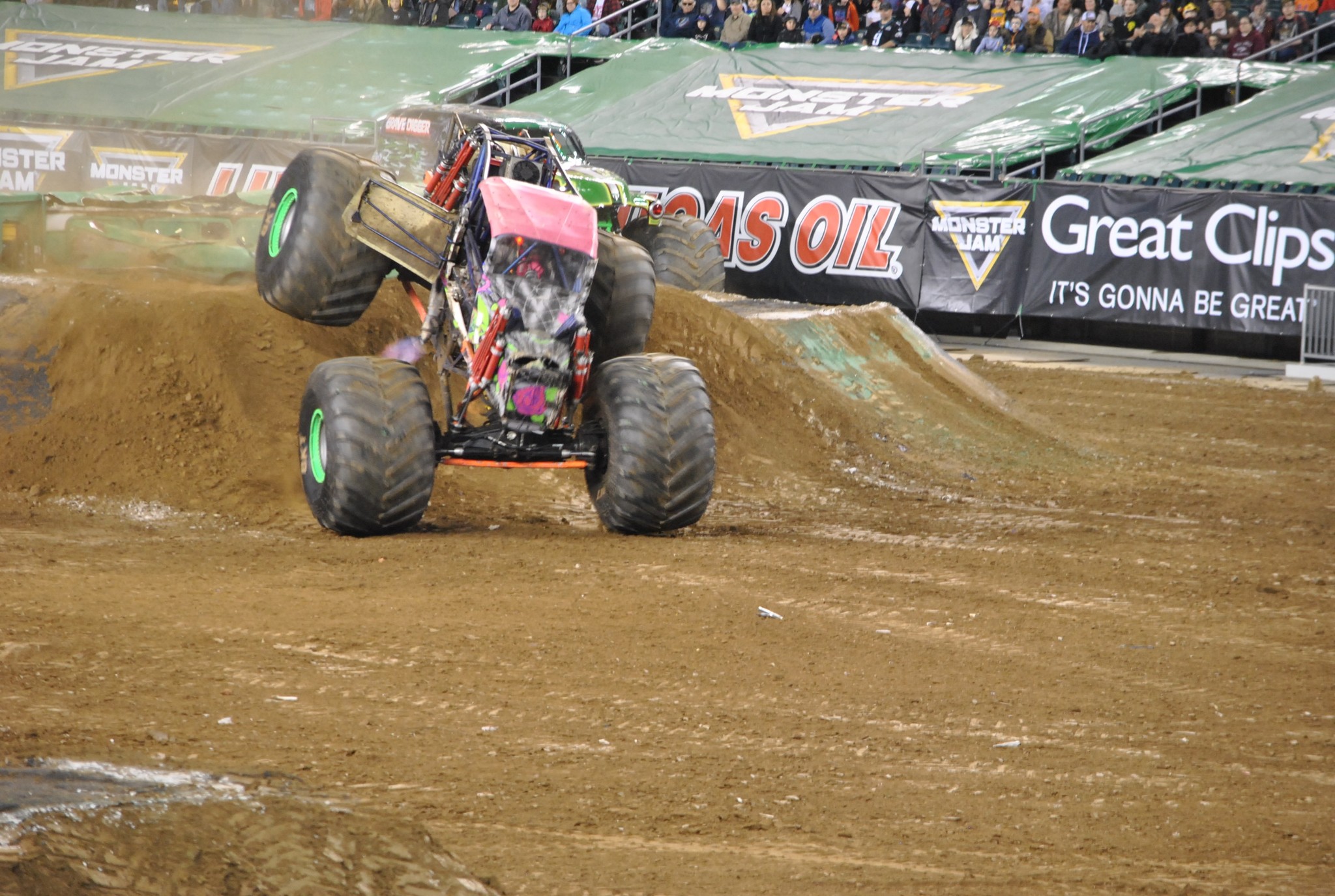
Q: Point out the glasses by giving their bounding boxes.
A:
[682,1,694,6]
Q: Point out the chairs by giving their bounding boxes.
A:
[1229,0,1335,42]
[851,29,955,50]
[445,13,503,31]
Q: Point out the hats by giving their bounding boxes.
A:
[808,3,821,12]
[694,15,706,23]
[1183,3,1198,16]
[731,0,743,7]
[989,20,1001,30]
[1082,11,1097,24]
[879,3,893,12]
[960,16,975,30]
[835,20,851,32]
[1028,5,1041,15]
[782,15,800,29]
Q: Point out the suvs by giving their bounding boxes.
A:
[254,110,718,538]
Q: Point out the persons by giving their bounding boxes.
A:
[45,0,1335,67]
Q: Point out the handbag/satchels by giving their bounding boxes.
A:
[811,34,824,44]
